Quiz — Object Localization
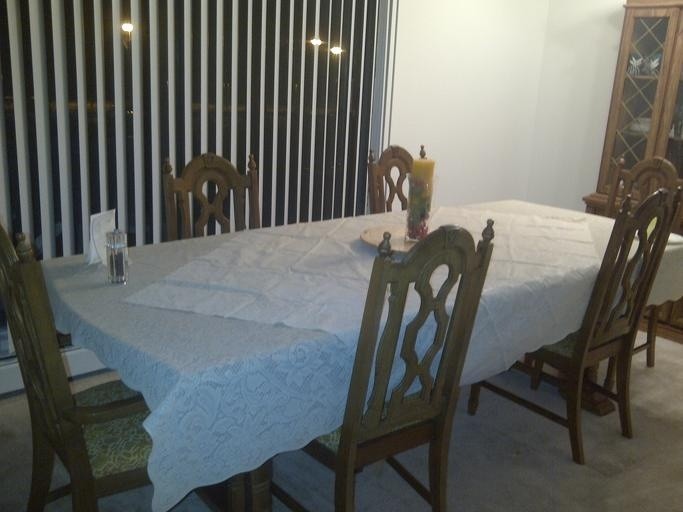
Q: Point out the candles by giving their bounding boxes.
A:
[404,153,437,239]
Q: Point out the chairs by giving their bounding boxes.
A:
[161,147,260,241]
[271,215,497,508]
[467,146,677,471]
[2,225,151,510]
[362,145,420,214]
[604,152,676,229]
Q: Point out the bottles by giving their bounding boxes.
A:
[105,229,128,287]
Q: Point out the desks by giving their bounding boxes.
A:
[30,195,683,510]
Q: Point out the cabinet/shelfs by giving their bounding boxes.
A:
[580,1,683,347]
